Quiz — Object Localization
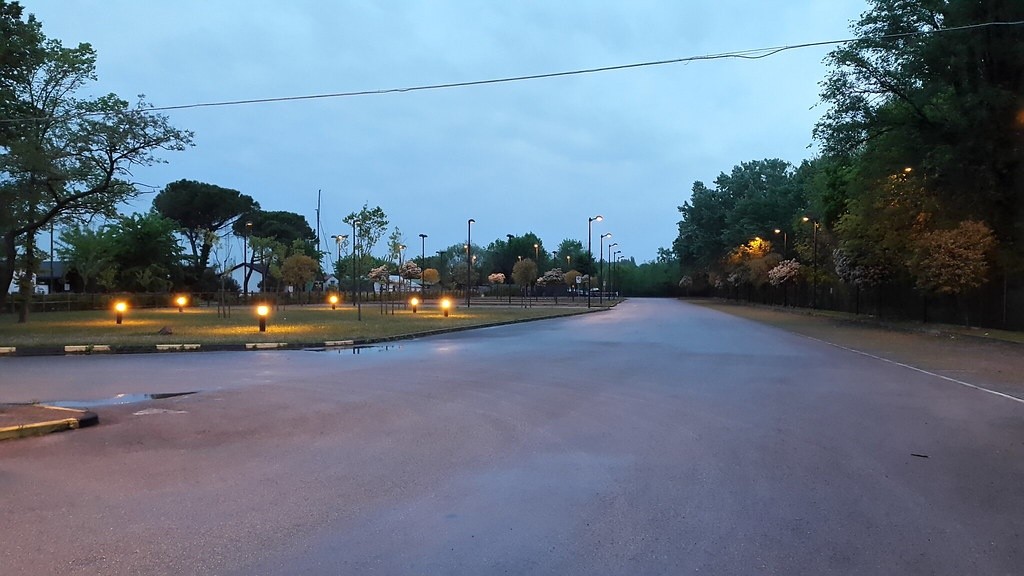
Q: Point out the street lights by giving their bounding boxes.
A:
[552,251,558,269]
[244,221,252,304]
[534,244,539,264]
[352,220,361,307]
[398,245,406,288]
[588,215,603,308]
[48,206,54,294]
[518,256,521,261]
[468,219,474,307]
[332,235,348,300]
[506,234,514,303]
[567,256,570,271]
[600,232,611,303]
[464,244,468,253]
[618,256,624,301]
[608,243,618,301]
[418,234,428,302]
[804,217,816,313]
[614,251,621,301]
[775,229,787,260]
[435,251,447,289]
